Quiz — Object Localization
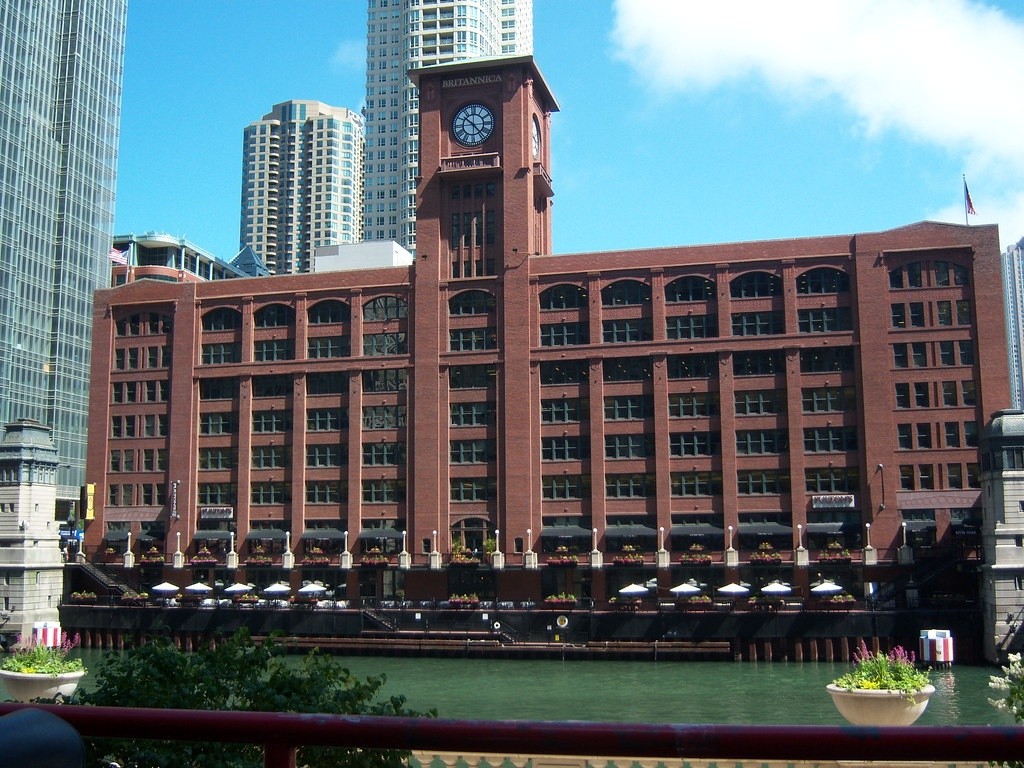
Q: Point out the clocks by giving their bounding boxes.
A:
[453,103,495,147]
[531,118,541,160]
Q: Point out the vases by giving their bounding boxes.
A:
[0,667,89,703]
[71,597,97,605]
[825,683,936,727]
[678,558,711,566]
[449,601,479,611]
[135,562,166,567]
[119,597,202,606]
[545,600,643,611]
[361,562,388,569]
[750,558,852,567]
[546,561,644,568]
[747,600,858,612]
[192,562,215,568]
[303,562,329,568]
[233,599,318,609]
[246,562,272,568]
[689,601,713,612]
[448,561,480,569]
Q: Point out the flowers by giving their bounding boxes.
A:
[189,556,218,562]
[832,638,933,706]
[70,589,96,598]
[244,555,273,564]
[0,630,89,679]
[362,555,390,565]
[449,592,478,600]
[139,555,166,562]
[288,595,319,603]
[120,589,203,602]
[300,555,330,564]
[749,552,782,562]
[817,549,851,560]
[687,595,712,601]
[748,594,855,602]
[544,591,643,603]
[450,554,481,563]
[548,554,643,564]
[231,592,259,601]
[679,553,712,563]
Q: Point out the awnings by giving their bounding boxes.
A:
[806,522,849,536]
[671,523,724,537]
[737,522,793,537]
[357,527,403,539]
[301,527,346,541]
[246,529,287,541]
[604,523,658,538]
[540,525,592,539]
[192,530,236,541]
[134,530,164,542]
[101,531,130,541]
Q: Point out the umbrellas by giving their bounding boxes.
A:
[152,581,180,606]
[619,582,648,608]
[263,584,291,608]
[670,582,701,600]
[223,583,253,591]
[298,584,328,611]
[810,583,843,611]
[761,582,792,613]
[185,582,212,608]
[716,582,749,597]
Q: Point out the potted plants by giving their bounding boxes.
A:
[104,548,117,560]
[198,546,212,558]
[253,545,265,559]
[555,544,635,556]
[308,547,324,558]
[367,546,382,558]
[689,543,705,557]
[482,534,496,564]
[827,541,843,555]
[759,542,775,556]
[395,589,406,602]
[147,547,159,557]
